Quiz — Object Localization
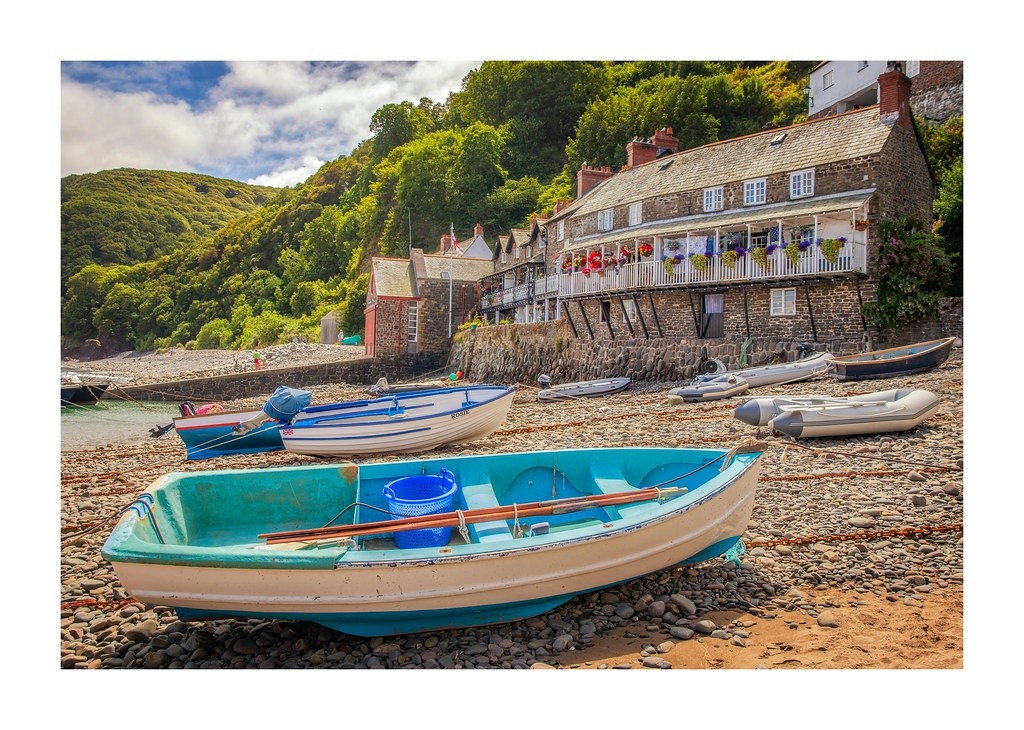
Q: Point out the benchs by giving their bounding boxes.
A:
[459,472,512,544]
[591,464,660,520]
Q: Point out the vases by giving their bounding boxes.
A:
[856,225,866,231]
[585,272,590,277]
[599,271,604,276]
[792,231,802,237]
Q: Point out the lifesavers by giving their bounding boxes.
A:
[704,361,717,372]
[701,362,705,372]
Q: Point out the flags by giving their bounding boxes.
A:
[451,225,463,256]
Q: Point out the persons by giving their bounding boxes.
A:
[376,371,388,386]
[338,329,343,345]
[263,359,269,369]
[493,279,499,291]
[254,350,260,370]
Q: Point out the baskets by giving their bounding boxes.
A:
[382,468,457,549]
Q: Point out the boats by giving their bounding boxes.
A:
[669,377,751,403]
[61,379,111,406]
[690,351,836,389]
[824,337,958,380]
[172,384,520,453]
[730,388,940,439]
[103,452,768,636]
[537,377,634,406]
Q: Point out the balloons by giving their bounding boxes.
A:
[450,371,456,381]
[456,370,463,380]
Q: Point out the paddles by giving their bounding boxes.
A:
[256,483,689,545]
[731,396,886,412]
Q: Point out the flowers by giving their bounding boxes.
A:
[748,244,778,266]
[716,230,745,268]
[639,244,653,256]
[779,241,810,266]
[560,244,632,274]
[660,240,684,277]
[816,236,846,263]
[850,219,869,226]
[784,226,813,233]
[688,251,713,270]
